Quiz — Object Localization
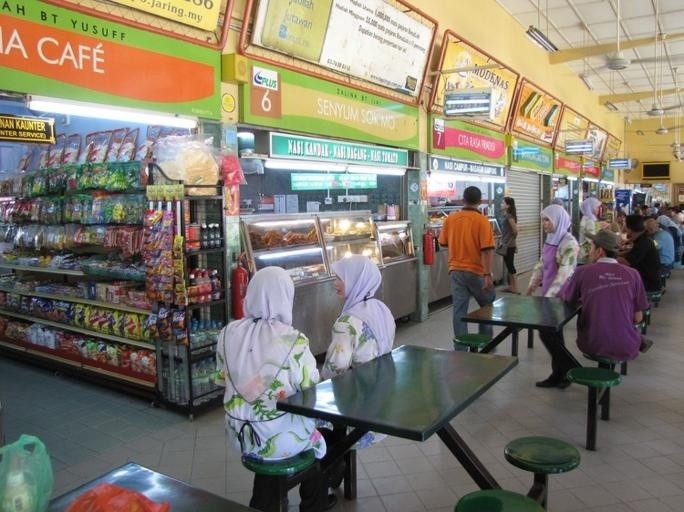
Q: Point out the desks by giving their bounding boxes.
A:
[460,293,584,374]
[275,343,521,491]
[43,461,257,511]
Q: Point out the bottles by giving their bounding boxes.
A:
[160,356,224,408]
[186,223,226,356]
[1,468,35,511]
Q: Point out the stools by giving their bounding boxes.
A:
[451,488,546,512]
[503,436,580,512]
[582,352,622,423]
[453,333,493,353]
[632,271,671,336]
[241,448,319,512]
[566,366,621,450]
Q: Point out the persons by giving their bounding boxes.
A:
[436,186,496,353]
[554,177,569,199]
[315,254,396,490]
[522,205,584,388]
[499,196,518,293]
[554,229,654,361]
[576,196,684,293]
[215,265,338,511]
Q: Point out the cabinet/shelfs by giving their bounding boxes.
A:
[0,163,231,422]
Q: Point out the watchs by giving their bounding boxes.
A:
[484,272,494,277]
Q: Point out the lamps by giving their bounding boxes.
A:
[442,86,497,119]
[526,0,684,171]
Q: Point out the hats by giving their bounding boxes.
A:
[583,229,619,253]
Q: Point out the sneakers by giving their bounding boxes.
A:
[328,457,346,489]
[535,360,583,390]
[300,493,336,511]
[640,338,654,354]
